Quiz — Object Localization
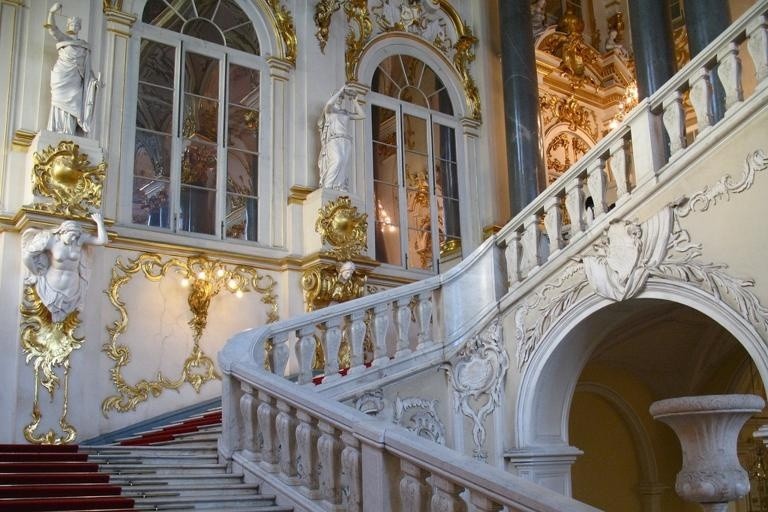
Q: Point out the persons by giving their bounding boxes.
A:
[44,3,102,136]
[605,31,628,55]
[339,260,356,281]
[22,212,109,323]
[531,0,546,34]
[316,84,366,189]
